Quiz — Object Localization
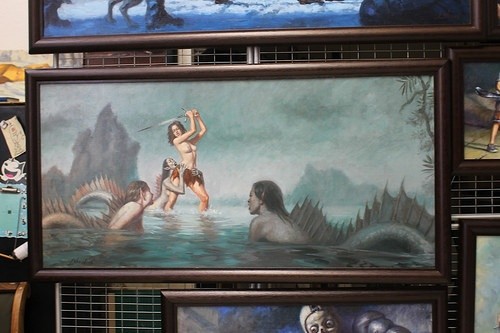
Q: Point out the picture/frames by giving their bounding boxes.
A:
[160,287,449,333]
[24,58,454,285]
[27,0,494,56]
[458,217,500,333]
[448,47,500,172]
